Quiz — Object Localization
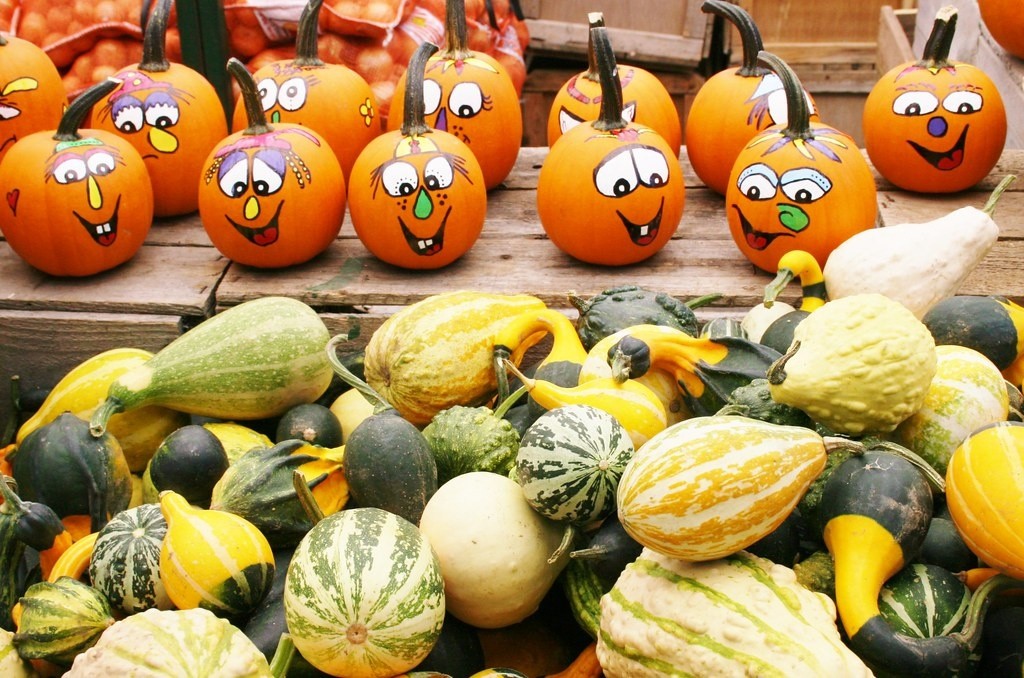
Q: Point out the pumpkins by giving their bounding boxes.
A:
[0,0,1024,678]
